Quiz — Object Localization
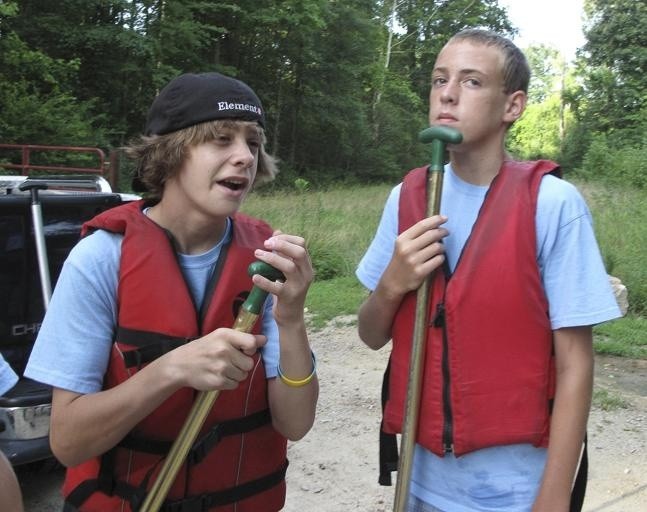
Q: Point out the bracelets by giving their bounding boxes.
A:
[276,345,317,389]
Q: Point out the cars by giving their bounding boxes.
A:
[1,176,145,468]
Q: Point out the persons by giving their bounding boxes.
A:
[0,353,30,512]
[22,70,320,512]
[354,29,624,512]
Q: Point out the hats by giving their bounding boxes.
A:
[130,71,266,193]
